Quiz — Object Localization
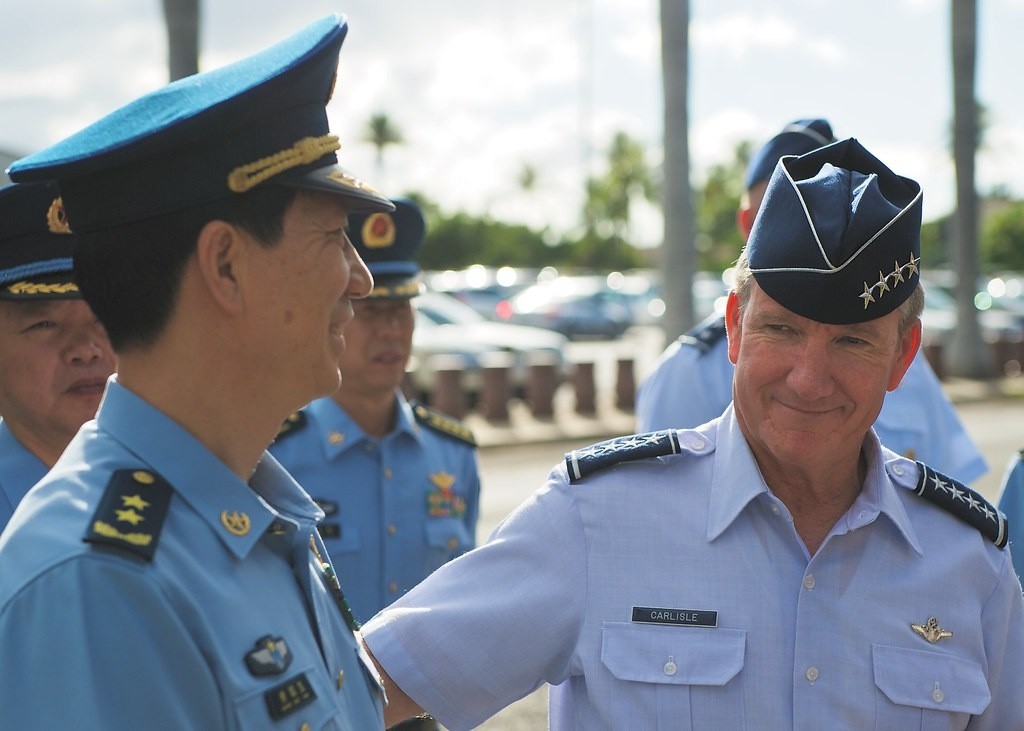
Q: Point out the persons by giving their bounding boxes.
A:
[0,8,396,731]
[355,138,1024,731]
[264,201,484,731]
[0,184,118,541]
[632,119,989,488]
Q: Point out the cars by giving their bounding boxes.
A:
[919,274,1024,365]
[505,277,665,339]
[408,297,568,399]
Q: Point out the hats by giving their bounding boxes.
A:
[746,136,923,326]
[744,117,839,190]
[4,13,397,236]
[341,196,426,302]
[0,183,82,300]
